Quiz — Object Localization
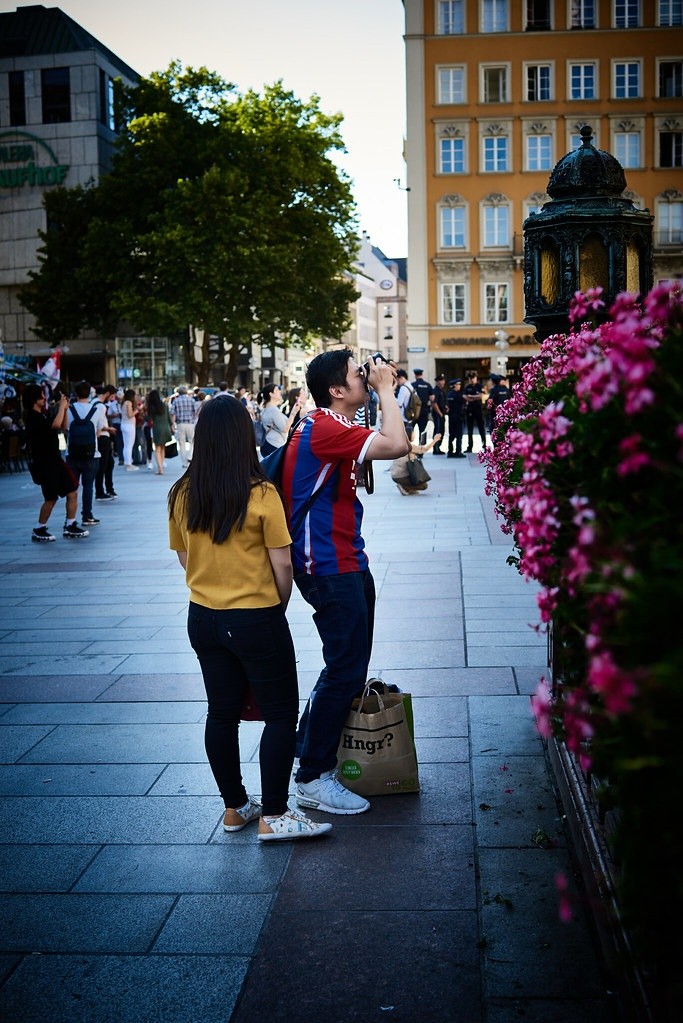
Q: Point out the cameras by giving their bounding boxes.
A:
[357,353,388,377]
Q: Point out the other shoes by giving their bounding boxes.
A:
[82,518,100,526]
[154,469,164,475]
[108,492,118,498]
[125,465,139,472]
[397,482,408,496]
[95,493,113,501]
[148,463,153,470]
[432,447,472,458]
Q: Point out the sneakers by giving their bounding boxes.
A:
[294,770,370,815]
[222,794,263,833]
[62,521,90,538]
[31,526,56,542]
[257,806,334,840]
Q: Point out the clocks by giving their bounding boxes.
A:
[379,279,393,290]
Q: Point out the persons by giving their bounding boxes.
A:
[0,381,319,543]
[168,397,334,843]
[283,351,412,818]
[352,369,518,495]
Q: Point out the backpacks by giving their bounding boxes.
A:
[401,384,421,421]
[259,408,330,539]
[68,403,97,465]
[253,409,274,447]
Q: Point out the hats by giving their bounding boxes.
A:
[466,370,506,384]
[433,374,445,382]
[178,386,188,394]
[450,378,462,385]
[413,368,424,375]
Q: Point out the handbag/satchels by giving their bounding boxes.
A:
[334,678,422,796]
[406,454,432,487]
[165,442,178,459]
[238,689,261,720]
